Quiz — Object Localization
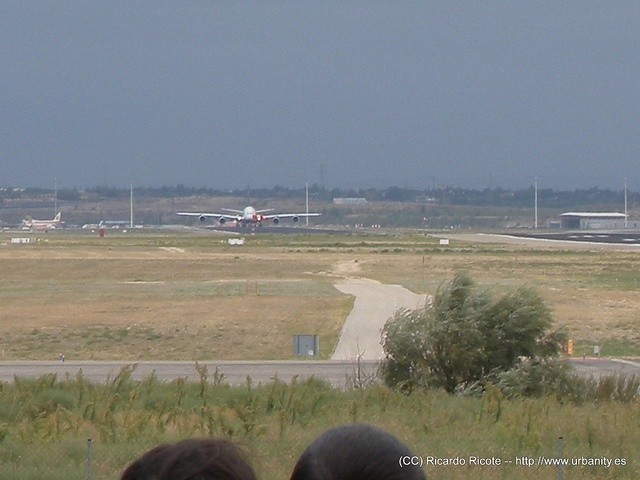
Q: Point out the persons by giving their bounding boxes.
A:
[290,424,427,479]
[119,438,256,480]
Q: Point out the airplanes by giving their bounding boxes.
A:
[176,206,321,227]
[82,220,119,232]
[22,211,65,232]
